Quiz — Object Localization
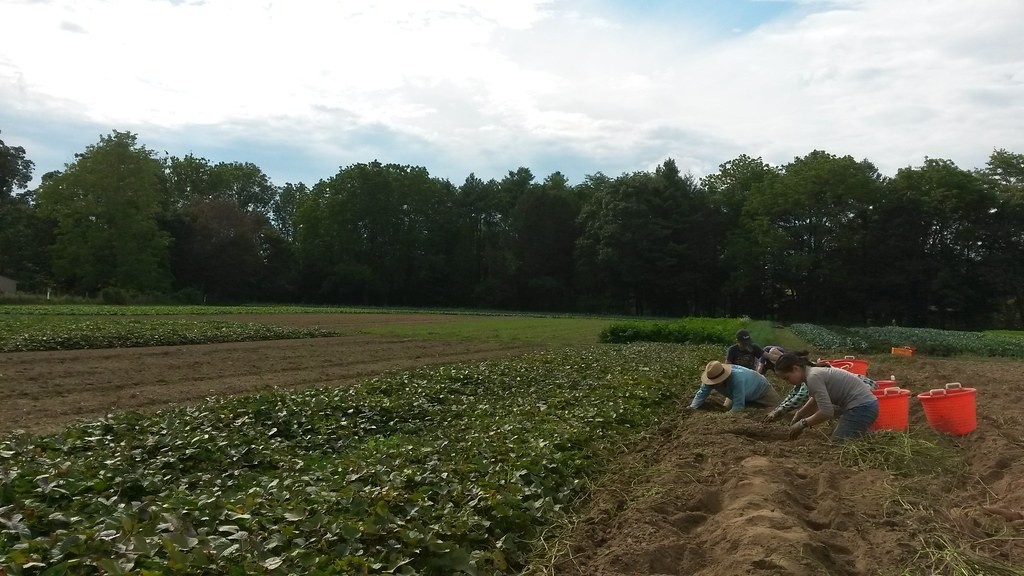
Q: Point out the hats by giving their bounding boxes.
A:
[736,330,750,341]
[701,360,731,385]
[769,348,783,364]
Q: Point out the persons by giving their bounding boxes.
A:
[686,329,879,445]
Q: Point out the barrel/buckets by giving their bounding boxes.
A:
[819,356,869,376]
[873,375,896,389]
[917,382,977,437]
[869,386,911,431]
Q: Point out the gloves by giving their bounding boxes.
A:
[789,412,804,426]
[789,419,807,440]
[761,410,776,425]
